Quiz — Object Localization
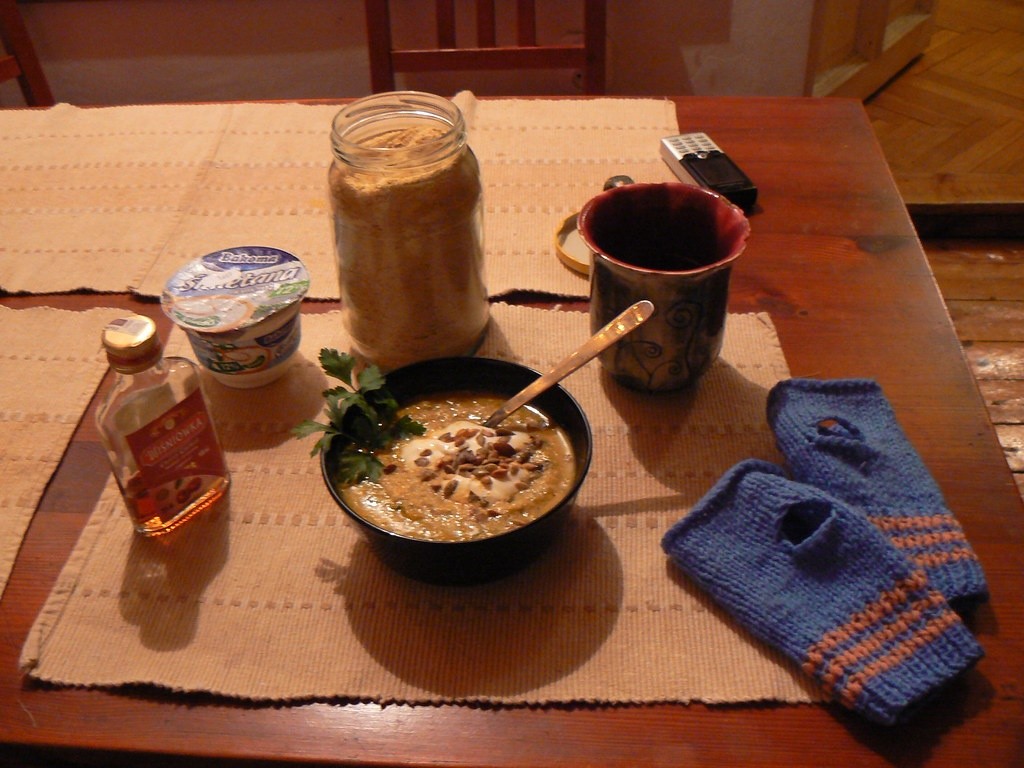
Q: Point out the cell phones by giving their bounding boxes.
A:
[660,132,757,206]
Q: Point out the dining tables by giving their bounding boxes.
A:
[1,93,1024,768]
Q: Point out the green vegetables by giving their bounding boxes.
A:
[288,348,427,485]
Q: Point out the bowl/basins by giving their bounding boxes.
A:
[321,356,593,572]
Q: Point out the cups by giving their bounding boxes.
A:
[577,175,750,394]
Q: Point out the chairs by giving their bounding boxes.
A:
[364,0,606,95]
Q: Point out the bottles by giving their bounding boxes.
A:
[328,91,489,369]
[94,316,231,538]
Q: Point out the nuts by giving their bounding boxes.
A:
[383,428,537,517]
